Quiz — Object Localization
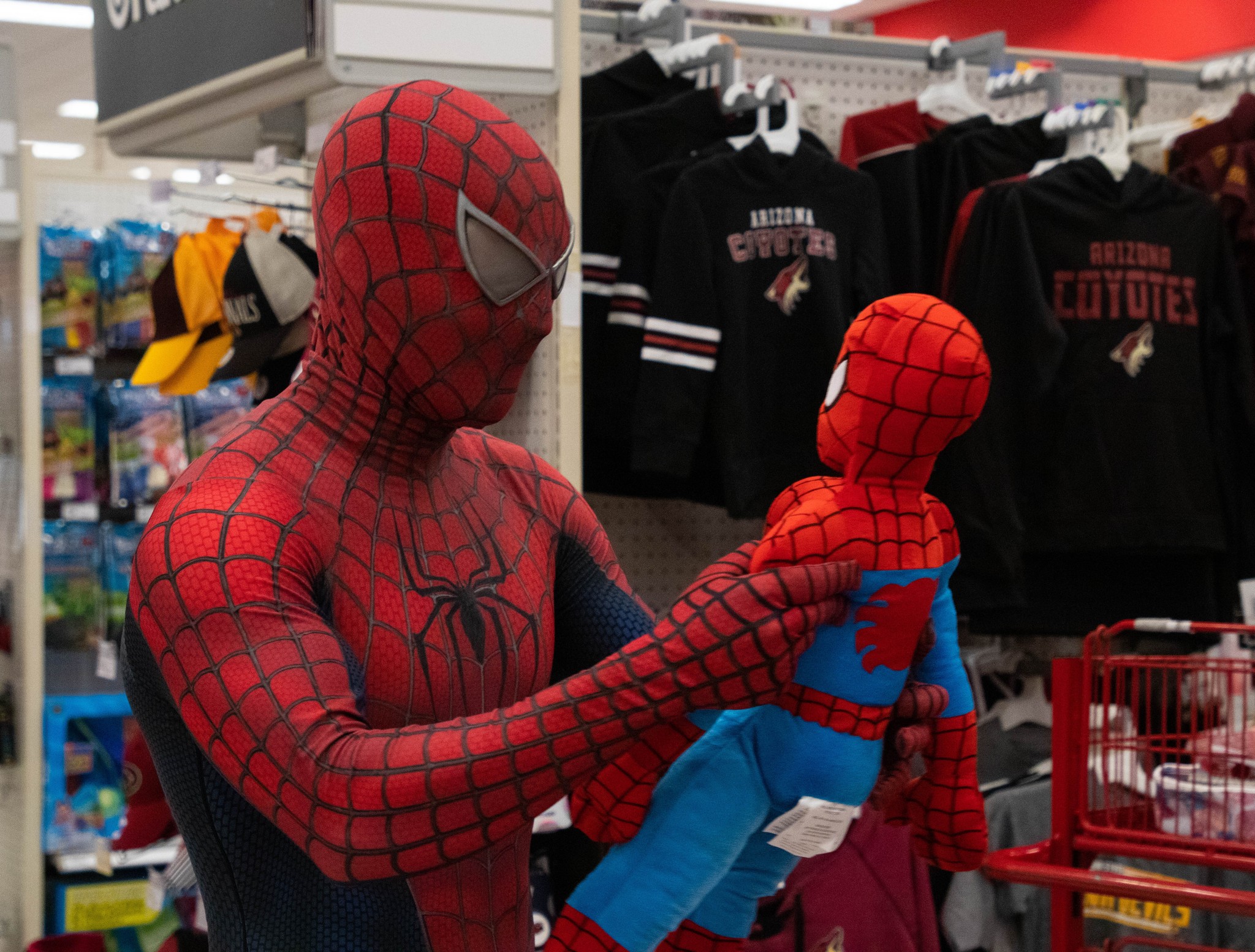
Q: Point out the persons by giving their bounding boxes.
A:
[543,293,989,952]
[124,80,949,952]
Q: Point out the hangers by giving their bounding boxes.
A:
[993,642,1156,798]
[917,37,1255,181]
[636,0,801,156]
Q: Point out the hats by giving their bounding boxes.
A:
[129,223,319,398]
